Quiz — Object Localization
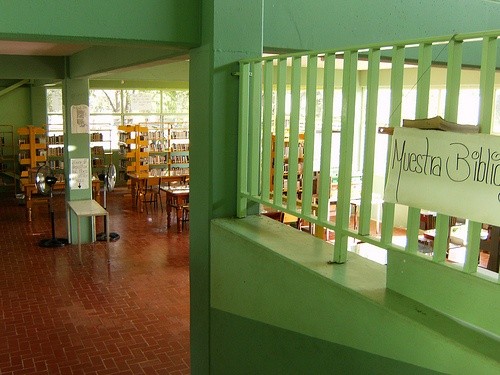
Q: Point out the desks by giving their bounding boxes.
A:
[67,199,111,264]
[21,176,101,223]
[160,185,190,233]
[326,195,493,276]
[126,169,189,213]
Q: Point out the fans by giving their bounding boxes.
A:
[35,164,69,248]
[96,163,120,241]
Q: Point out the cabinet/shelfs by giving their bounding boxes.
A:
[137,121,190,172]
[267,131,312,193]
[50,130,113,180]
[19,123,48,204]
[119,123,150,188]
[0,123,17,197]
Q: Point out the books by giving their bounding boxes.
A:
[91,146,105,156]
[419,213,457,232]
[283,140,304,157]
[403,115,480,134]
[35,160,64,171]
[282,162,304,173]
[140,168,191,179]
[37,135,65,144]
[282,176,304,191]
[118,159,132,169]
[118,131,131,143]
[50,173,66,181]
[139,155,190,164]
[93,160,104,166]
[416,233,466,253]
[17,150,29,161]
[91,132,103,142]
[16,135,28,146]
[140,142,189,153]
[119,146,132,156]
[38,146,65,159]
[18,167,28,178]
[142,129,191,140]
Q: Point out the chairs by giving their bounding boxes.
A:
[261,192,360,242]
[133,165,190,226]
